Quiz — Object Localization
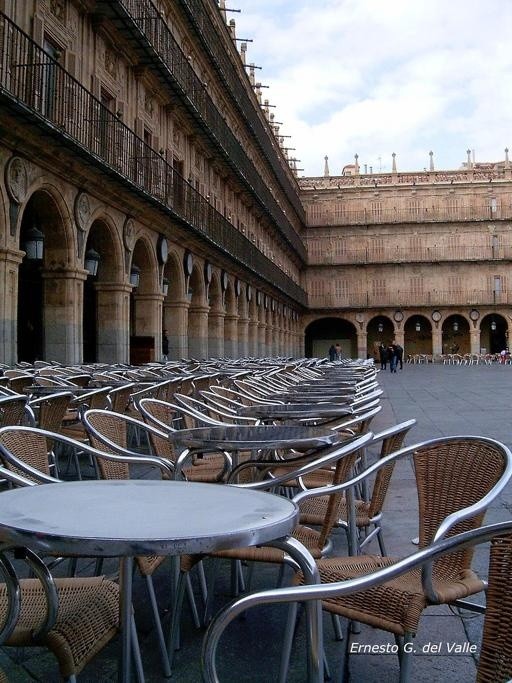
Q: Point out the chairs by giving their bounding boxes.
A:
[0,352,512,683]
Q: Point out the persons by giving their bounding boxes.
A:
[379,339,403,373]
[450,341,457,354]
[162,329,169,358]
[329,343,343,361]
